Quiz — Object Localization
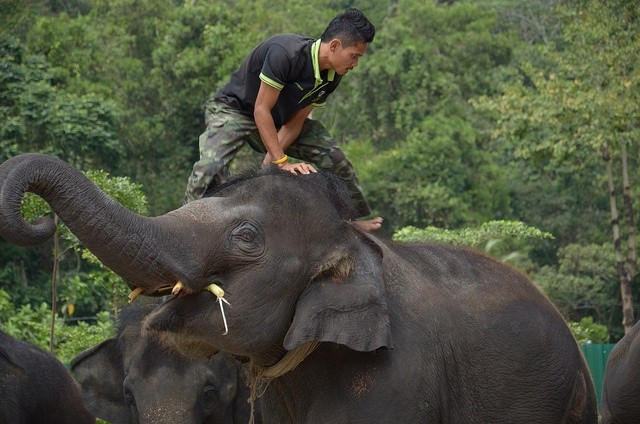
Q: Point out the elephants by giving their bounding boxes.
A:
[68,301,262,424]
[0,153,600,424]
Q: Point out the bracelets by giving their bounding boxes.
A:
[271,155,288,164]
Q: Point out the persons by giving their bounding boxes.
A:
[183,9,383,232]
